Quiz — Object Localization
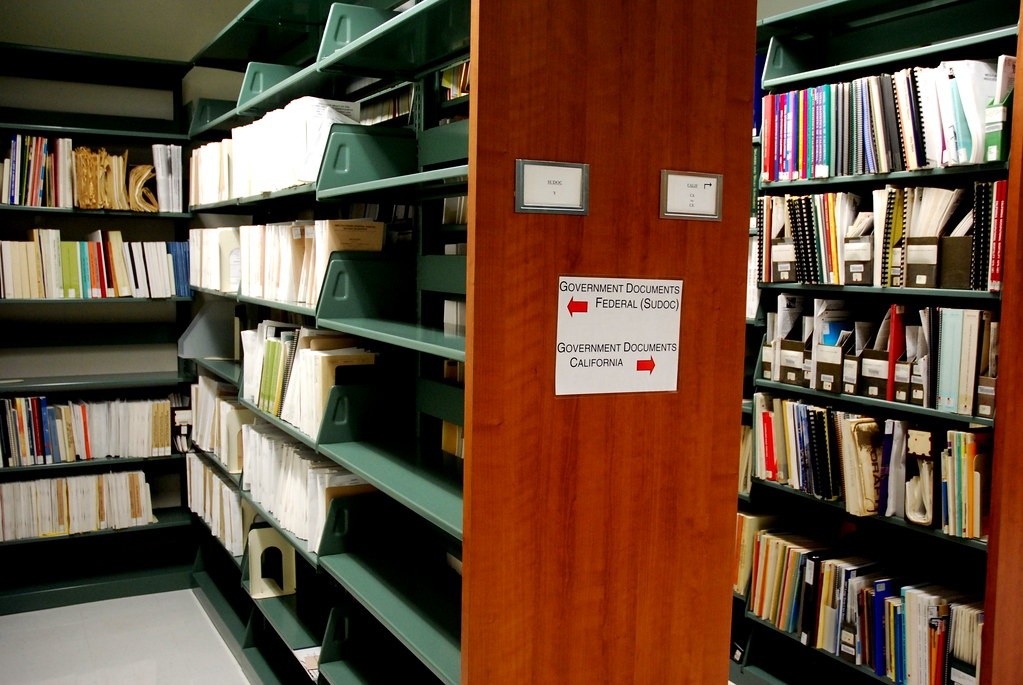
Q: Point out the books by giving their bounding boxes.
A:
[0,134,184,213]
[356,56,463,460]
[737,513,985,685]
[185,317,375,559]
[762,292,998,417]
[0,393,190,468]
[0,226,189,299]
[747,177,1007,323]
[0,471,152,542]
[750,53,1017,216]
[739,392,988,541]
[188,95,386,309]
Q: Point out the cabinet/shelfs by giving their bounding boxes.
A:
[175,0,757,685]
[731,0,1023,685]
[0,41,193,546]
